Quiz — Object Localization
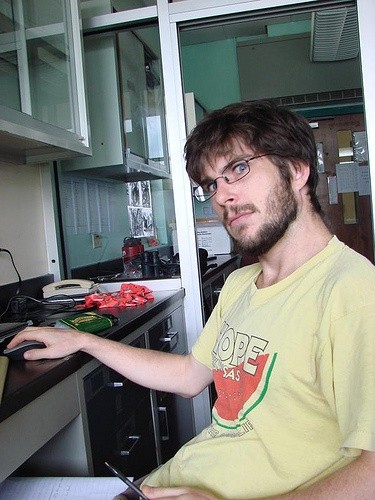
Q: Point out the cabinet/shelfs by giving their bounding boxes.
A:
[67,11,168,180]
[0,254,241,486]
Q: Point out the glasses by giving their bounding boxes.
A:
[191,152,277,203]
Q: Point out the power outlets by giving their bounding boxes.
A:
[92,233,103,249]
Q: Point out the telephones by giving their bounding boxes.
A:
[41,278,100,298]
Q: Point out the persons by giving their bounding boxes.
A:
[7,101,375,500]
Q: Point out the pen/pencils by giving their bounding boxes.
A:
[105,461,150,500]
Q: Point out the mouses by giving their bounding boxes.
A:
[3,339,47,361]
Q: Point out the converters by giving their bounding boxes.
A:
[10,297,28,314]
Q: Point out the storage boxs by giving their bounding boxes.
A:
[169,218,235,256]
